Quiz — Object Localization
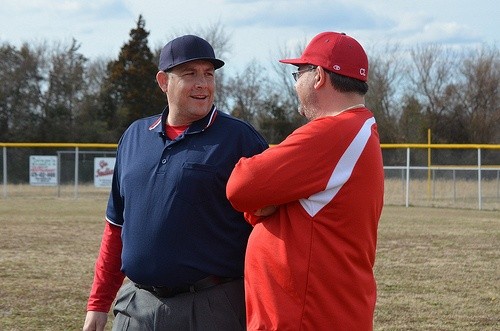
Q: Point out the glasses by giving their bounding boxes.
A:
[292,68,316,81]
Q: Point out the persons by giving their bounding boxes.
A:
[226,32,385,331]
[83,35,268,331]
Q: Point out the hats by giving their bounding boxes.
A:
[278,32,369,83]
[159,35,225,71]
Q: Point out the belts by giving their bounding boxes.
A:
[134,274,238,297]
